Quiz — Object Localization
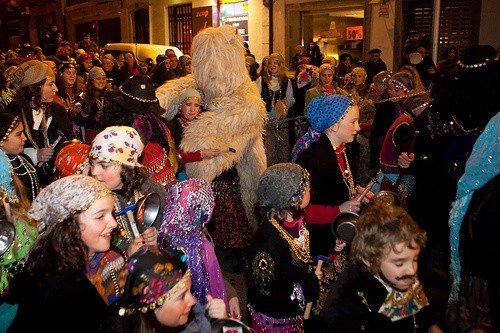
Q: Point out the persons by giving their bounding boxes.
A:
[87,126,160,258]
[0,24,244,288]
[249,162,320,333]
[156,177,249,333]
[125,244,217,333]
[308,202,445,333]
[244,40,432,204]
[0,174,144,333]
[397,30,500,333]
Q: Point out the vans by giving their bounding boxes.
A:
[104,43,184,65]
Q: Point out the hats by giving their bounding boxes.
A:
[484,44,497,58]
[408,28,422,36]
[368,48,382,54]
[118,74,158,103]
[454,46,493,79]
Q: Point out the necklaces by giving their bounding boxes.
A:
[265,214,312,263]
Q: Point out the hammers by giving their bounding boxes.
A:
[117,203,142,244]
[38,130,67,167]
[357,176,380,203]
[218,147,236,156]
[302,255,332,322]
